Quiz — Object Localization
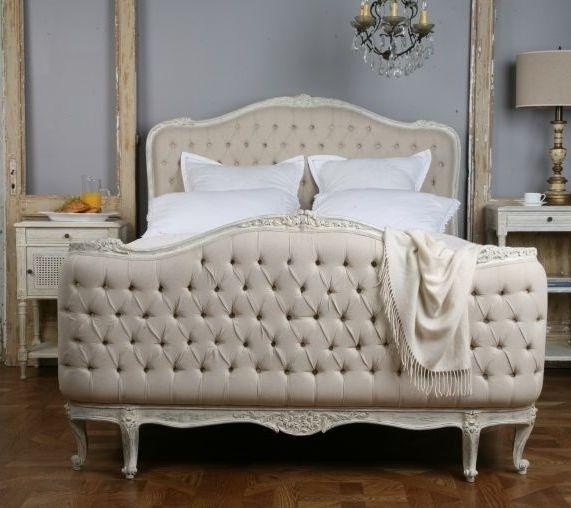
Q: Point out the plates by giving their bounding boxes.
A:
[37,210,120,222]
[521,201,547,207]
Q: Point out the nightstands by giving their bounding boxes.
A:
[13,221,125,380]
[485,203,571,363]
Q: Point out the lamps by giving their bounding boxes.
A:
[515,46,571,204]
[350,0,436,80]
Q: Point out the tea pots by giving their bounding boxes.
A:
[80,175,111,211]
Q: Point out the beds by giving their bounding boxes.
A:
[56,88,548,483]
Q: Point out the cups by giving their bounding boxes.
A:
[523,192,546,203]
